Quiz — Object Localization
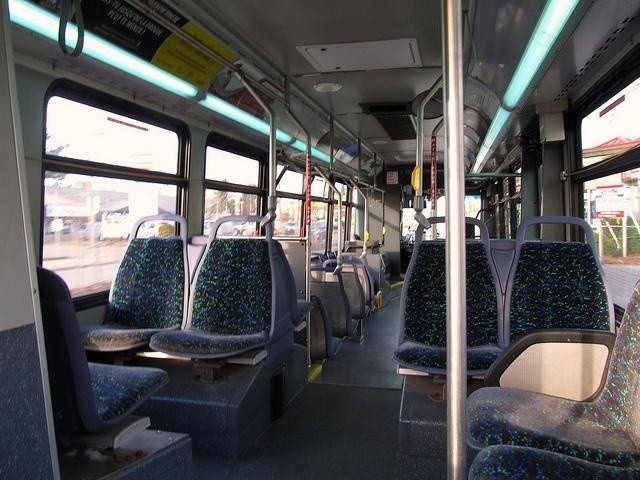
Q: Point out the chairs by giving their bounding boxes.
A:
[79,214,277,383]
[37,269,168,461]
[396,217,504,374]
[308,251,386,366]
[505,216,616,344]
[464,282,638,479]
[31,213,391,479]
